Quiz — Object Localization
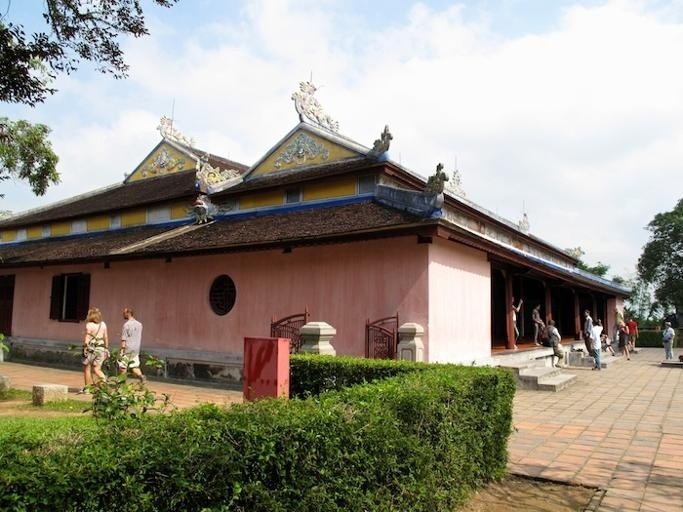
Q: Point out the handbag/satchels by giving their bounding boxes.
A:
[662,336,672,343]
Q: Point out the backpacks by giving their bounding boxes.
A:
[540,327,551,344]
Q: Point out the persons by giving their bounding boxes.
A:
[618,316,638,361]
[116,306,147,385]
[662,321,676,359]
[512,297,523,349]
[584,310,615,370]
[79,307,110,394]
[541,320,564,368]
[531,304,545,346]
